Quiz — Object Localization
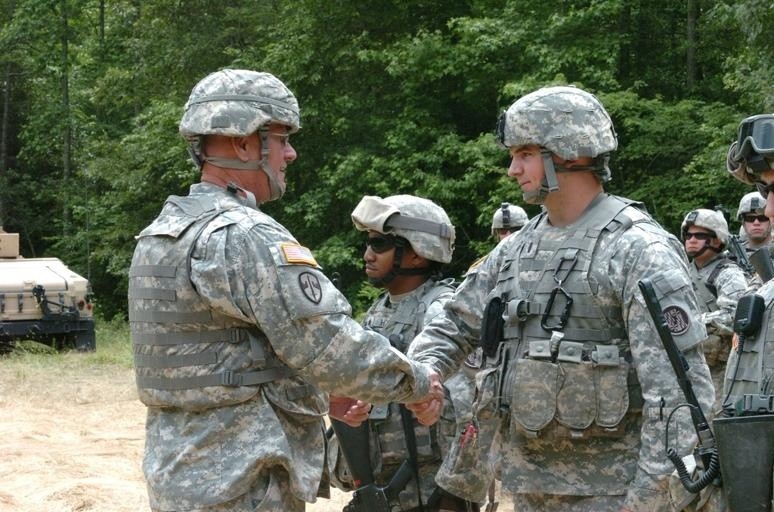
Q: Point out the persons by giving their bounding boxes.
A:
[680,207,751,409]
[327,194,488,512]
[128,70,445,510]
[465,201,531,276]
[736,191,774,291]
[404,82,717,510]
[668,114,774,512]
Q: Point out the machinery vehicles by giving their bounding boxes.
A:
[0,229,94,350]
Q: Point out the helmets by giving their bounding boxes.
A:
[735,192,764,219]
[679,208,727,245]
[351,192,455,266]
[503,85,617,160]
[178,65,302,141]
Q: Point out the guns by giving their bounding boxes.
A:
[714,202,756,274]
[747,245,773,283]
[634,280,724,494]
[329,411,417,512]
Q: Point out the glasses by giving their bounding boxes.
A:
[684,229,713,242]
[742,215,769,224]
[731,114,774,157]
[364,235,396,252]
